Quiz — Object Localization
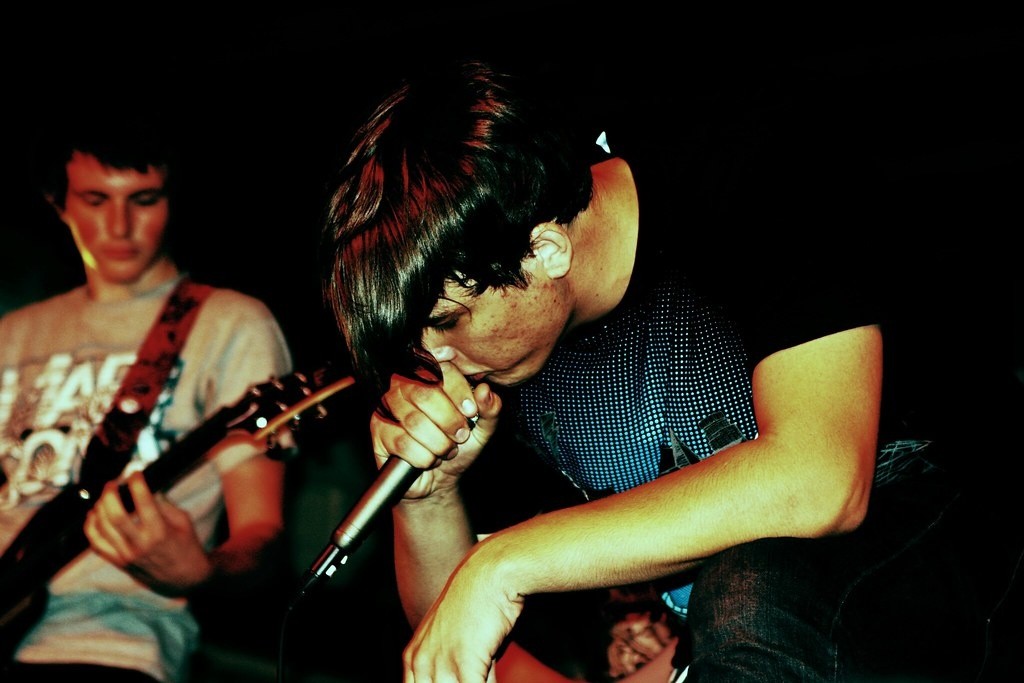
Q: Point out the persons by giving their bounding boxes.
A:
[322,63,945,683]
[1,96,294,683]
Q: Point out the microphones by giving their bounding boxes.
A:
[298,380,480,592]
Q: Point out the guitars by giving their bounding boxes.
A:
[1,354,358,677]
